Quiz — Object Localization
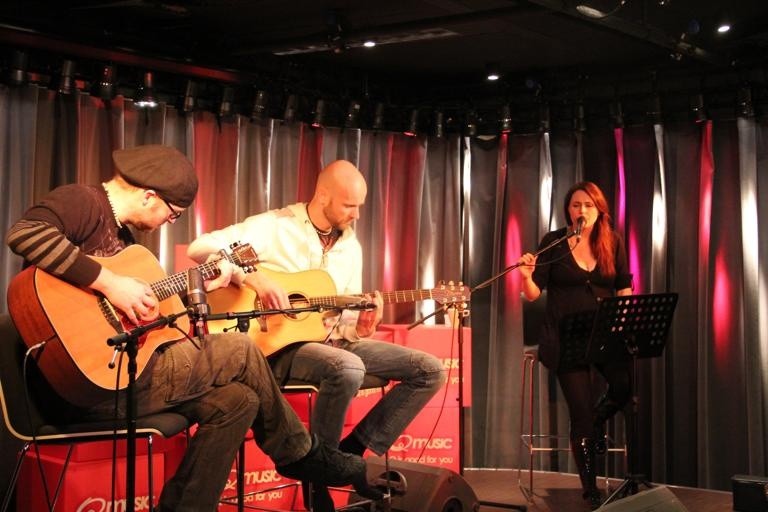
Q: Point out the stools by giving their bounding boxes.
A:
[519,346,628,501]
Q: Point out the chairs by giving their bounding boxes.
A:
[0,312,200,511]
[235,373,391,512]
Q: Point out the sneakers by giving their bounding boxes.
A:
[276,432,385,511]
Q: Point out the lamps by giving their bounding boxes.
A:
[576,0,630,19]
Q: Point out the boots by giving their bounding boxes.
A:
[570,433,601,509]
[593,392,618,455]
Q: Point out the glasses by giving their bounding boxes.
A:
[163,200,182,219]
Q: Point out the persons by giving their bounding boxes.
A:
[185,160,448,512]
[4,144,367,512]
[515,181,653,506]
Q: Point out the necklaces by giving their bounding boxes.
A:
[101,182,124,229]
[315,225,333,236]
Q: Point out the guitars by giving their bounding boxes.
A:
[202,265,472,356]
[7,239,260,407]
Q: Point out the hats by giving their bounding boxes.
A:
[113,146,198,207]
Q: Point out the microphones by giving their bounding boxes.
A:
[574,214,587,241]
[186,267,210,348]
[357,297,381,312]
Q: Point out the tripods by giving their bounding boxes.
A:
[588,352,680,508]
